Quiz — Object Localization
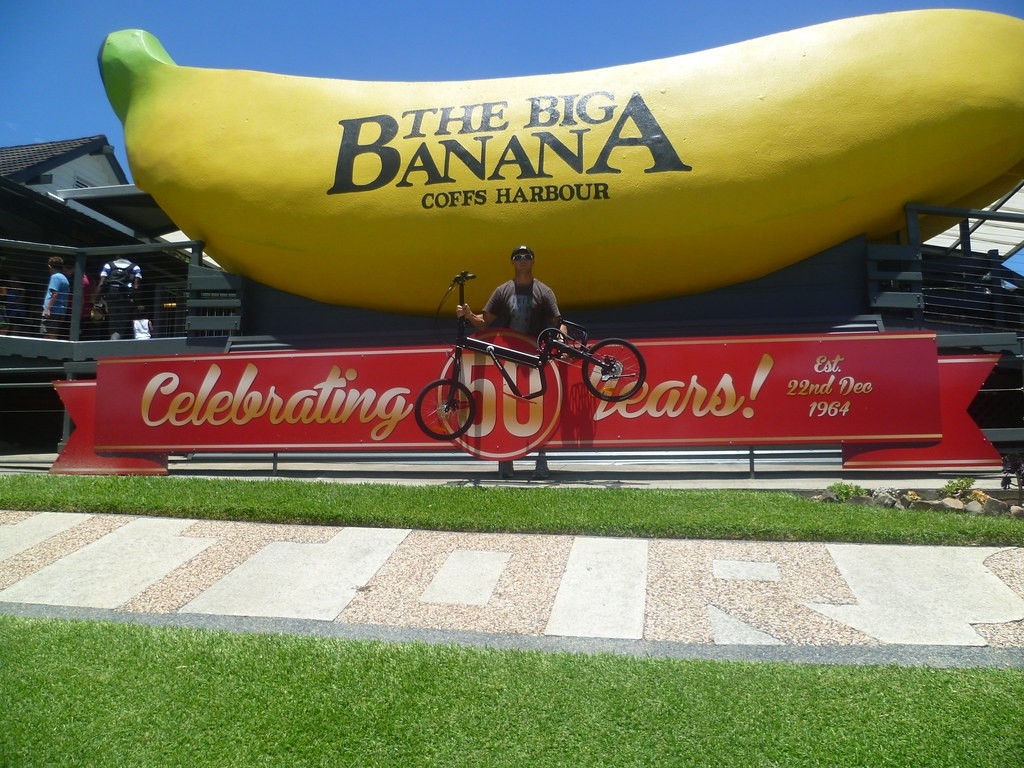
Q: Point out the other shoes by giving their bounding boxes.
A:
[536,459,550,478]
[501,461,515,478]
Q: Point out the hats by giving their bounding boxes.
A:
[511,245,534,260]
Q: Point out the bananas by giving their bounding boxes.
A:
[97,9,1023,316]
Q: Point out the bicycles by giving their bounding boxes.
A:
[415,271,647,439]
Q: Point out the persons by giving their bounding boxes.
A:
[0,253,155,340]
[455,245,567,479]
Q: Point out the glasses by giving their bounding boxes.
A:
[513,254,533,260]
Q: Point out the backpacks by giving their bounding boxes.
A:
[101,261,137,301]
